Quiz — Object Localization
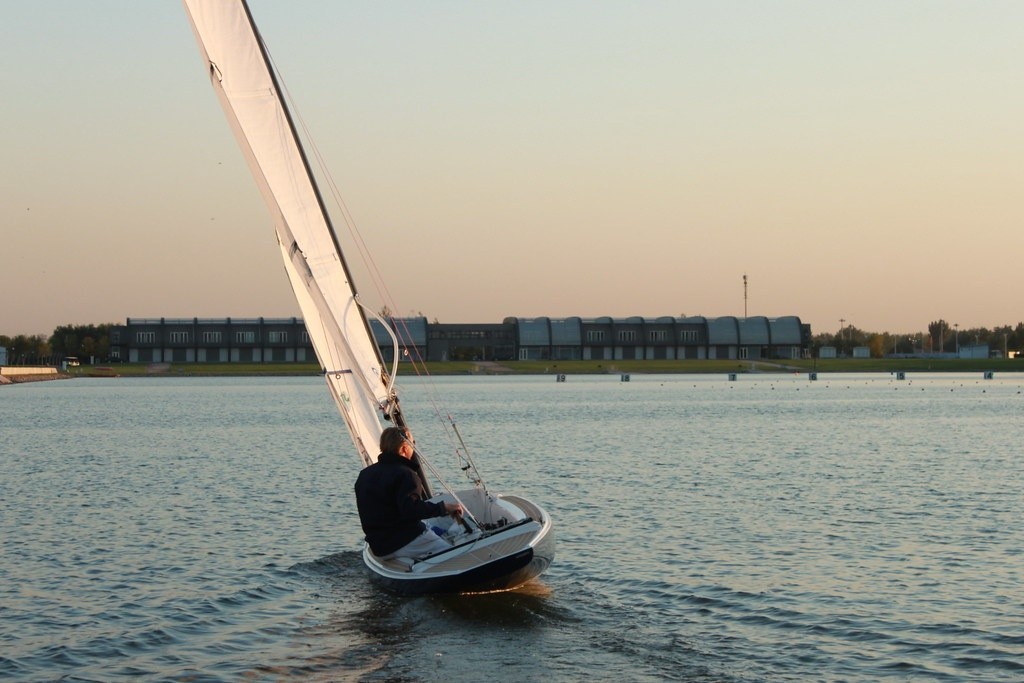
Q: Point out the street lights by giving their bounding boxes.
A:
[1003,333,1008,360]
[975,334,979,345]
[954,323,960,358]
[837,318,845,354]
[848,323,855,350]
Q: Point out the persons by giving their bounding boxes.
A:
[355,426,464,561]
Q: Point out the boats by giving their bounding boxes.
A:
[87,366,121,377]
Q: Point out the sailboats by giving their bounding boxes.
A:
[178,0,555,599]
